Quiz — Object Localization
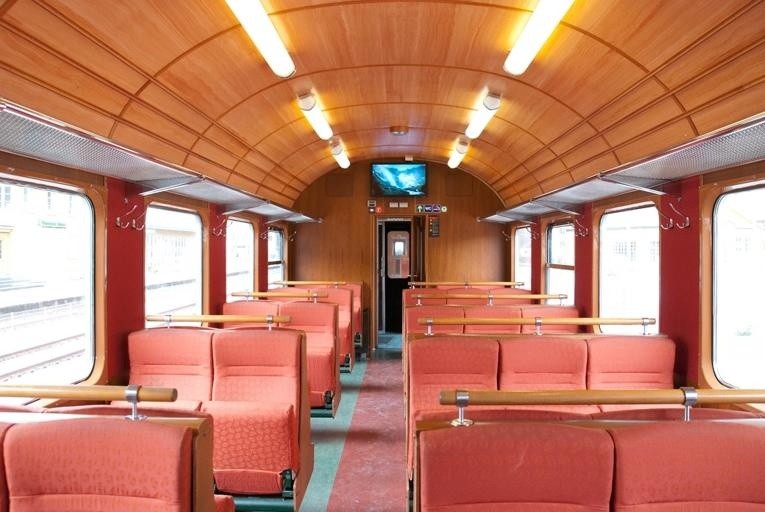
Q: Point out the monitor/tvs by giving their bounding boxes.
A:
[369,162,428,197]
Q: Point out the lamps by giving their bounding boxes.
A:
[464,92,501,139]
[299,91,334,141]
[226,0,296,77]
[330,141,350,169]
[447,139,469,169]
[501,0,573,76]
